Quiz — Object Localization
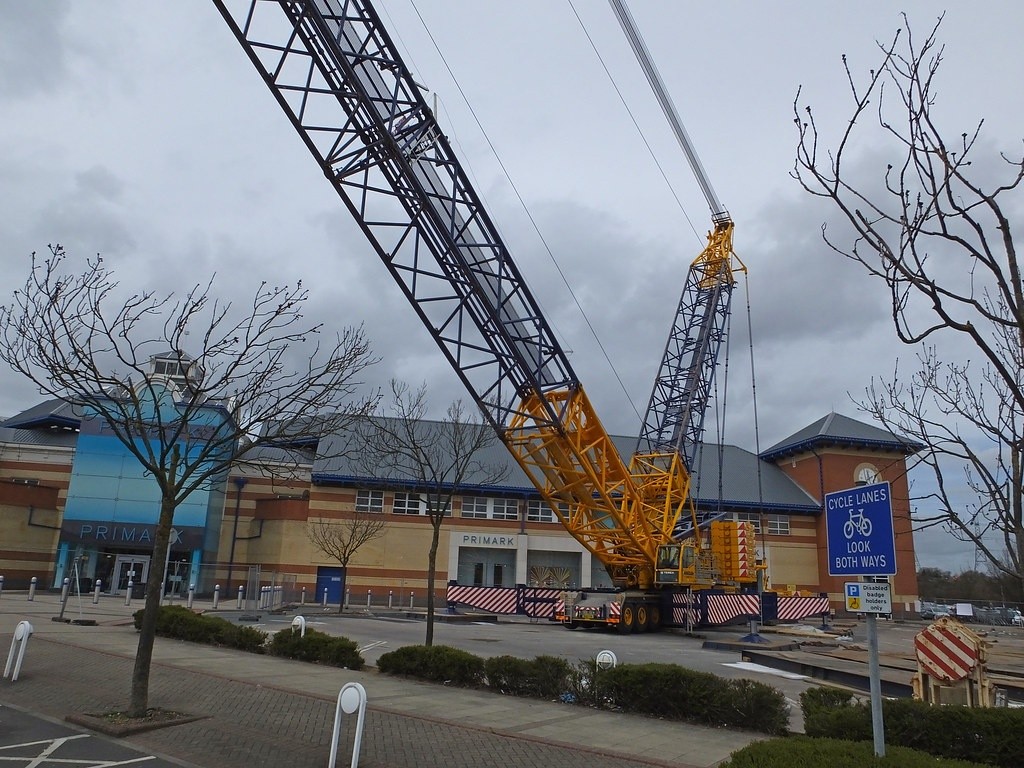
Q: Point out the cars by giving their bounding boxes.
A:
[921,601,1024,627]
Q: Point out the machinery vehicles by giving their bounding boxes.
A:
[202,0,778,635]
